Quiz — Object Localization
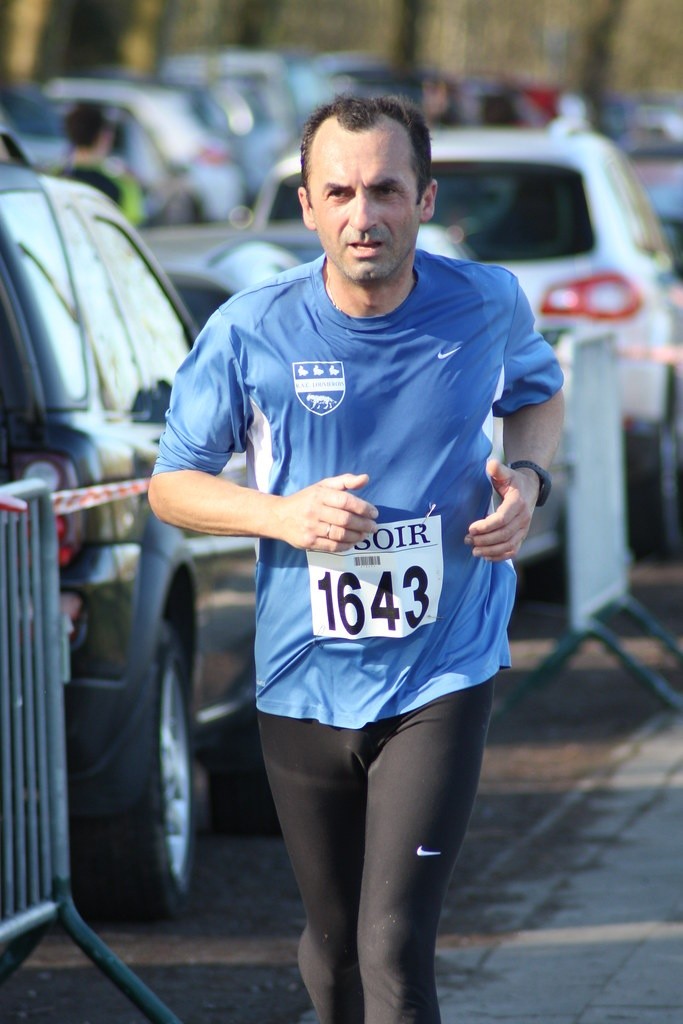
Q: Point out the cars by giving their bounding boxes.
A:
[0,45,683,614]
[0,175,280,924]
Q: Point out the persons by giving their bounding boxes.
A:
[38,99,148,228]
[148,94,564,1024]
[421,72,556,127]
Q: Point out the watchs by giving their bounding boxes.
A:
[508,460,552,507]
